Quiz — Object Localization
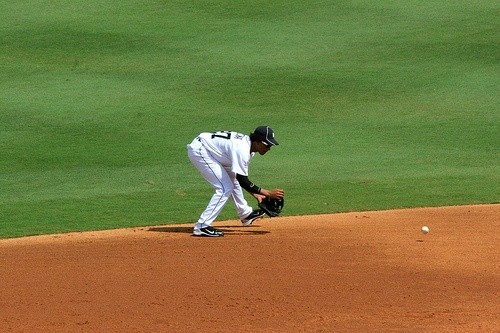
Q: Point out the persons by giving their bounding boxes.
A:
[186,126,285,237]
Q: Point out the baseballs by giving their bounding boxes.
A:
[421,226,430,234]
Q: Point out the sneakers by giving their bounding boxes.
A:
[241,209,265,226]
[193,225,224,237]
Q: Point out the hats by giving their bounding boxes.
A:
[254,126,279,146]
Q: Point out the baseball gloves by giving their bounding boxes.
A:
[258,196,284,217]
